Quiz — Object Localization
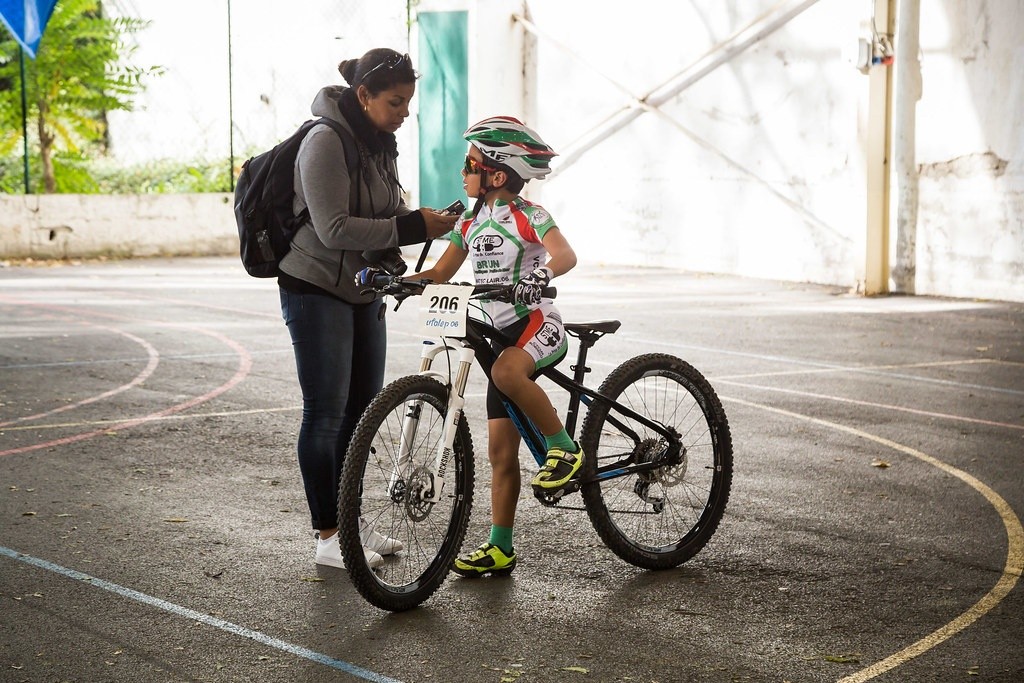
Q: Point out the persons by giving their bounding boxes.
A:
[354,116,585,579]
[276,49,461,570]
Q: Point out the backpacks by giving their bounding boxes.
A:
[235,118,357,278]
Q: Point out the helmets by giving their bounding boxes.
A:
[463,117,558,182]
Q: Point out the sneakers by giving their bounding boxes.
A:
[451,542,518,579]
[533,441,588,490]
[315,518,402,569]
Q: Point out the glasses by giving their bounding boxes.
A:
[466,154,494,176]
[361,52,413,85]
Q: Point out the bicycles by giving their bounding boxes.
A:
[334,265,734,610]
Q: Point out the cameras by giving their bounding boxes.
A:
[362,246,408,277]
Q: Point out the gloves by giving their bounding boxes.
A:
[512,267,554,306]
[354,267,394,289]
